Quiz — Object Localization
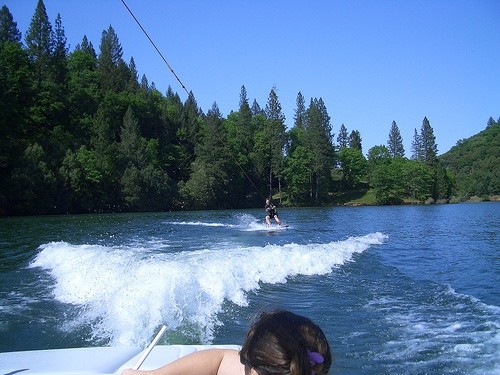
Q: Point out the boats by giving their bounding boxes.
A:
[0,345,247,375]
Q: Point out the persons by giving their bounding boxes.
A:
[120,307,332,375]
[265,198,282,227]
